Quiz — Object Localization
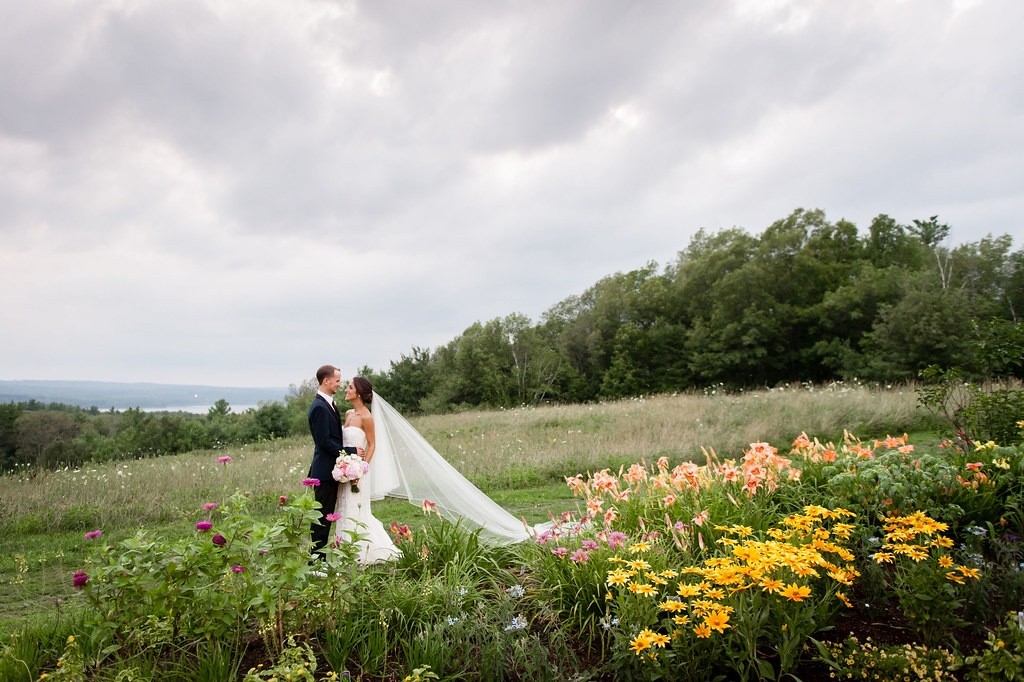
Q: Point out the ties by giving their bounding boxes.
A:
[332,401,340,420]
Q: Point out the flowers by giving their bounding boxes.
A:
[331,449,369,492]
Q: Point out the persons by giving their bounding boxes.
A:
[308,365,366,568]
[335,378,404,565]
